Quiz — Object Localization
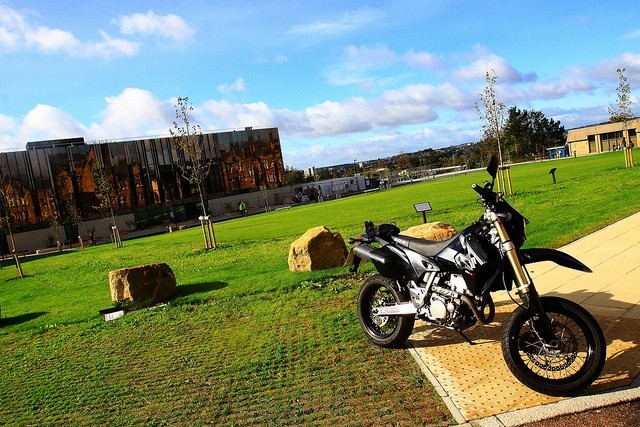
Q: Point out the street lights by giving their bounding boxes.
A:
[353,159,360,191]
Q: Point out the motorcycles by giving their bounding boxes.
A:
[342,154,606,396]
